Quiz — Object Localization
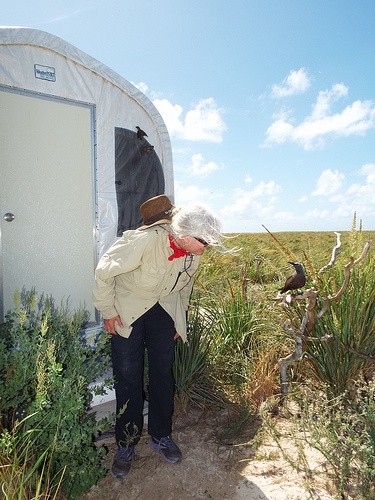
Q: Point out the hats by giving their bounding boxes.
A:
[136,194,182,231]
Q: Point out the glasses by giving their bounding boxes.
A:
[193,236,210,248]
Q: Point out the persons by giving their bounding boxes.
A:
[94,206,243,478]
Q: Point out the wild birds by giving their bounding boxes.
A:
[278,261,306,294]
[144,146,154,152]
[135,126,148,141]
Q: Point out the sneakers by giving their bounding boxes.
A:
[149,434,182,464]
[111,445,135,479]
